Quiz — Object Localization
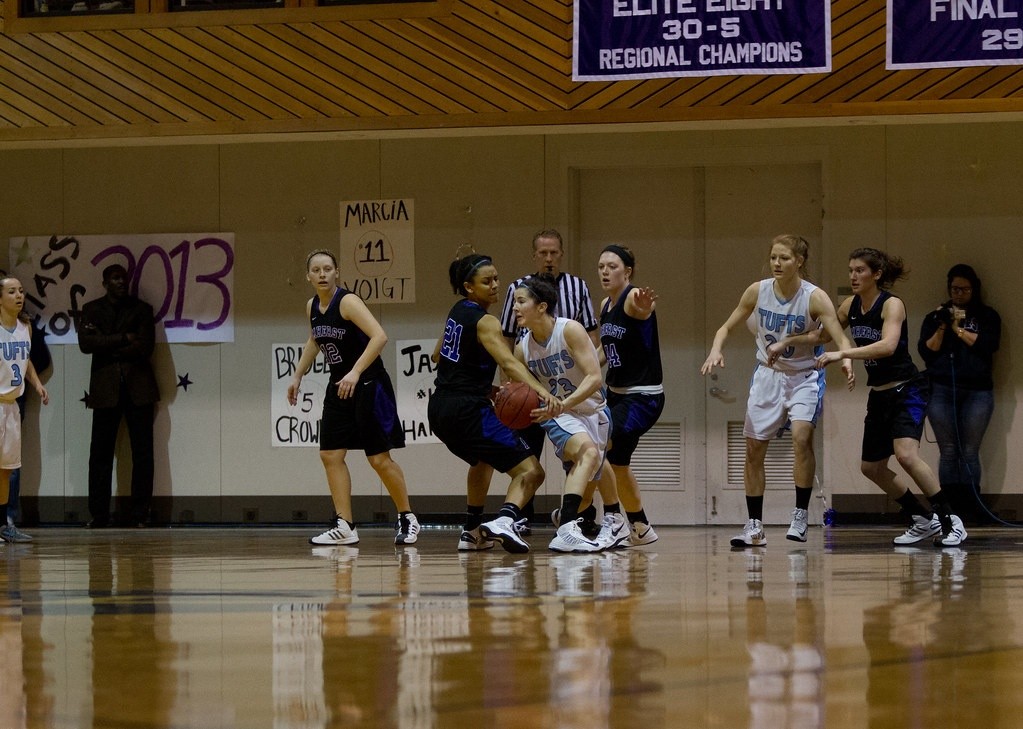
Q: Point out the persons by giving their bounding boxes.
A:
[496,279,613,553]
[593,244,665,550]
[0,277,50,542]
[768,248,968,546]
[287,249,421,545]
[78,264,157,529]
[500,230,602,538]
[701,234,856,547]
[427,254,563,553]
[0,269,51,527]
[917,264,1001,528]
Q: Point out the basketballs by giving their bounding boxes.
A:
[494,382,540,430]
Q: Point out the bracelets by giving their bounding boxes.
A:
[958,328,965,338]
[939,327,945,330]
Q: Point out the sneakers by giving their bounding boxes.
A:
[311,546,359,561]
[394,545,417,567]
[786,507,808,542]
[312,513,360,544]
[892,512,941,545]
[615,521,658,548]
[479,516,531,553]
[786,550,808,580]
[394,511,420,544]
[551,506,601,537]
[731,519,767,547]
[548,517,605,552]
[594,511,630,547]
[0,517,32,543]
[933,548,967,574]
[458,523,494,550]
[934,514,967,545]
